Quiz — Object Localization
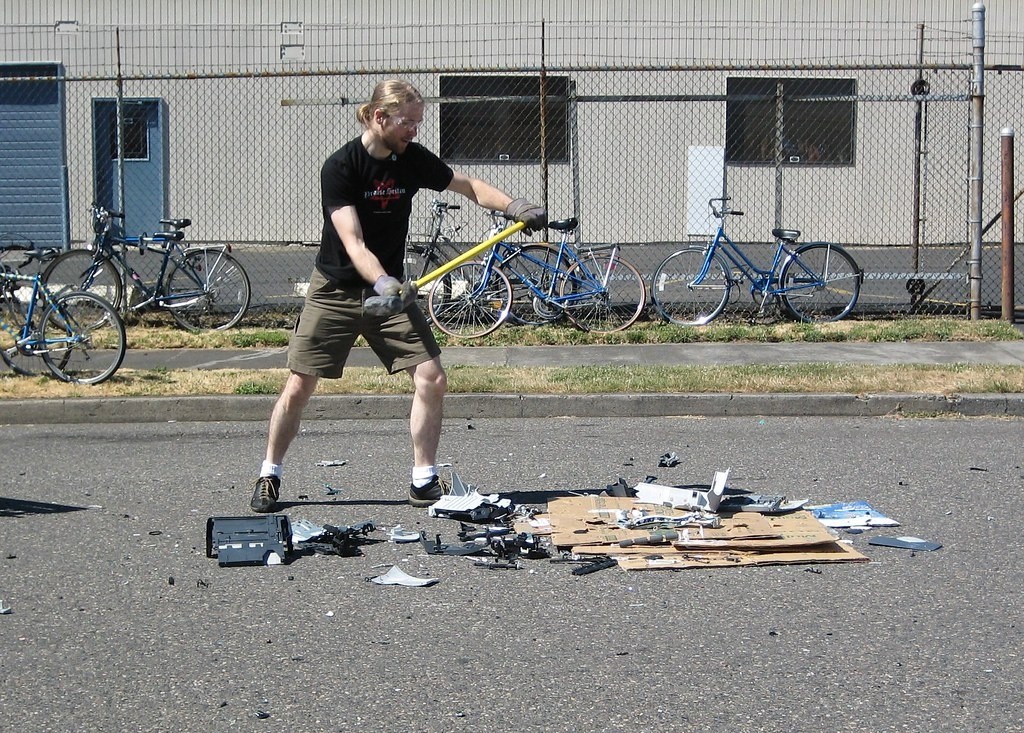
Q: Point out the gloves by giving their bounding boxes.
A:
[503,197,549,237]
[373,276,419,309]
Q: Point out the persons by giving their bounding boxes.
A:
[250,76,548,514]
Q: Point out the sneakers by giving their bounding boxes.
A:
[408,475,452,507]
[249,476,280,513]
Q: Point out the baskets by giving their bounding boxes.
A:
[687,232,715,253]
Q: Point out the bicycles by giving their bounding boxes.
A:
[403,197,647,339]
[0,200,251,386]
[650,196,863,326]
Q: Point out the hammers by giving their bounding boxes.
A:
[364,220,528,317]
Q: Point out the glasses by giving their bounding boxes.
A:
[387,114,423,130]
